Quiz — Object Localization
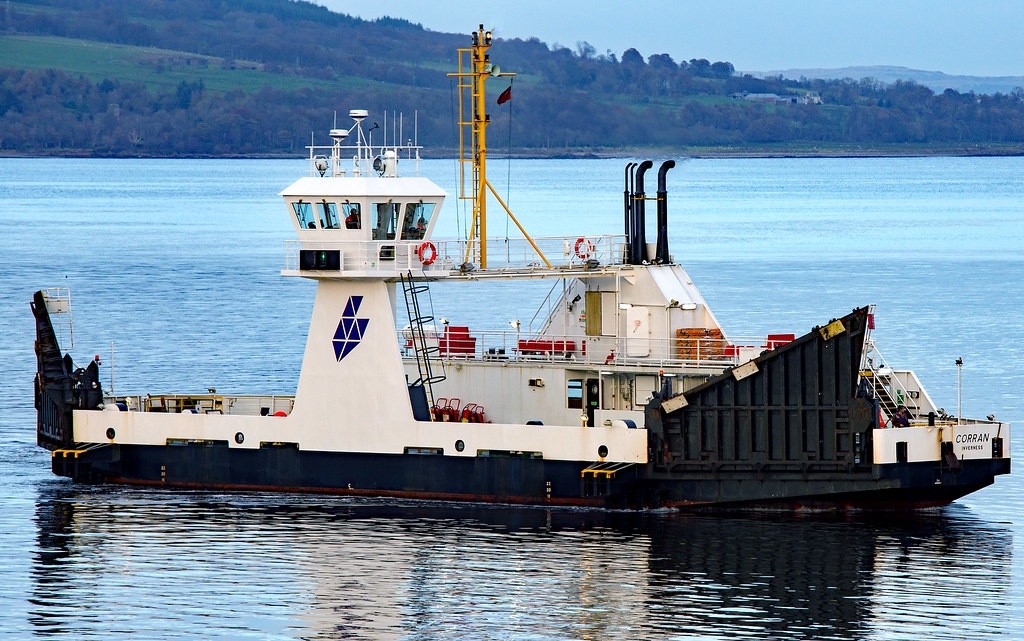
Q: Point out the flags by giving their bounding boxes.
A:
[497,85,514,105]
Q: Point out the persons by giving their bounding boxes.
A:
[417,218,428,239]
[345,208,361,229]
[892,405,910,428]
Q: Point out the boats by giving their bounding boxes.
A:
[30,25,1011,518]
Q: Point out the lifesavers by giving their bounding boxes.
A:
[418,242,437,265]
[574,238,592,258]
